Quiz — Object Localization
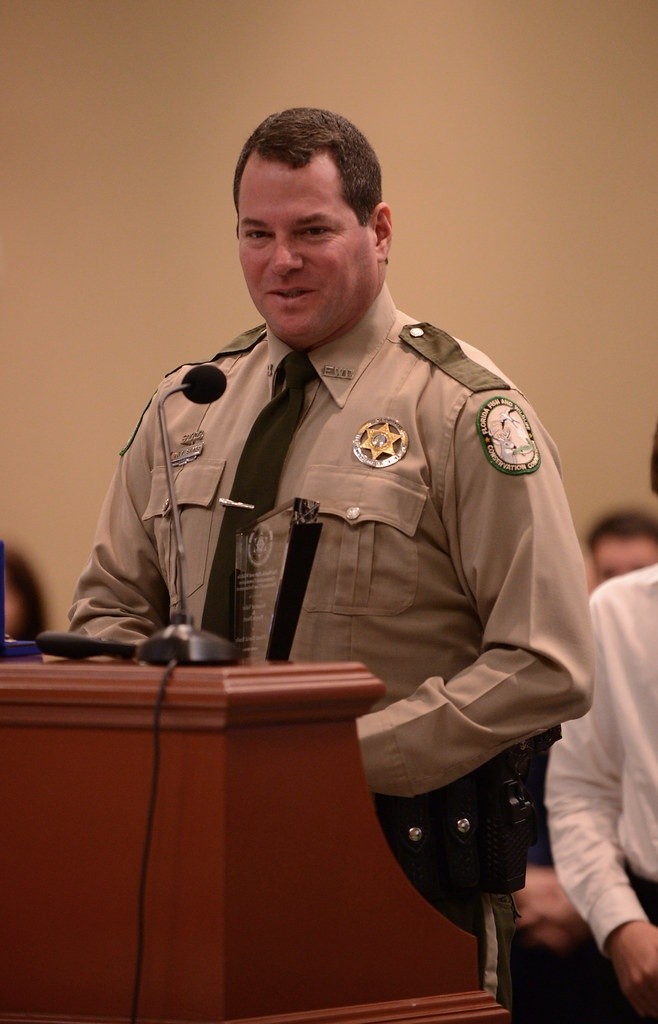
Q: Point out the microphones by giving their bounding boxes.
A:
[136,365,243,665]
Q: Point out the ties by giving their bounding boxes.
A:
[200,350,319,644]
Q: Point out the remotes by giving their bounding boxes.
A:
[35,631,137,661]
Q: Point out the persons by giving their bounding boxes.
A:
[4,555,44,641]
[495,513,658,1024]
[543,420,658,1024]
[66,107,597,991]
